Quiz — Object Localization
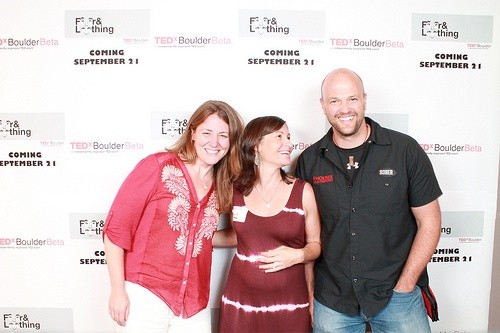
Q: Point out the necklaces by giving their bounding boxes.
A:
[252,179,283,208]
[188,163,208,190]
[334,123,368,166]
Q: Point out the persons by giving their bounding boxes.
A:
[293,68,443,333]
[102,100,244,333]
[212,116,321,333]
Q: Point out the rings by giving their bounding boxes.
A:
[271,264,275,270]
